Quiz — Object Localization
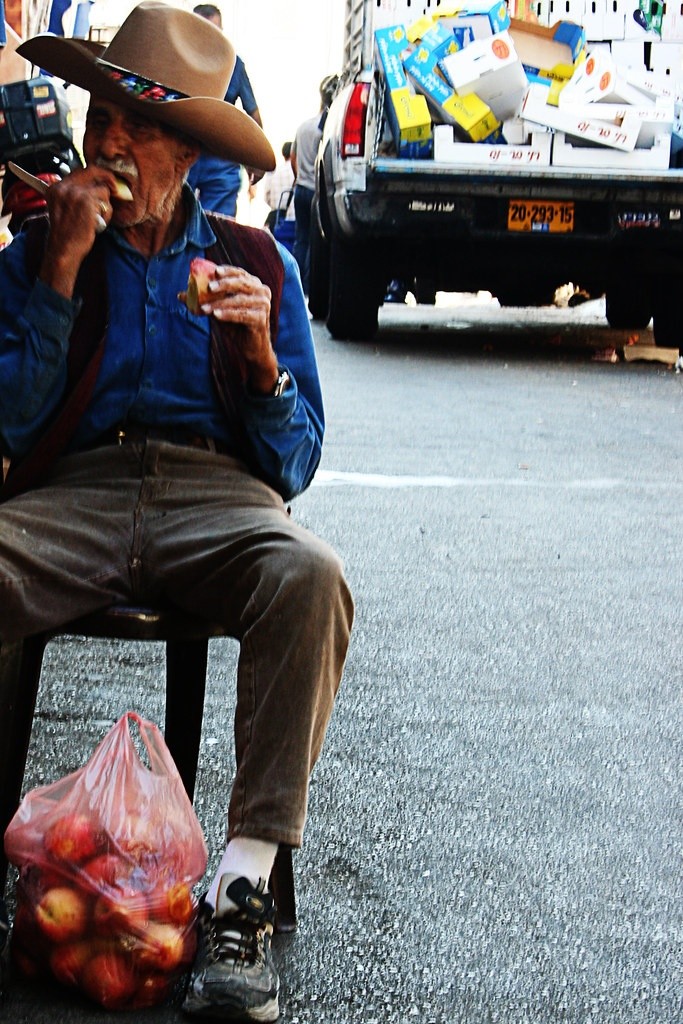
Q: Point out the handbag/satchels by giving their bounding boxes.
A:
[4,711,209,1010]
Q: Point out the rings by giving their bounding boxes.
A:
[98,201,108,212]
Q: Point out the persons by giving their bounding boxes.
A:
[0,0,354,1024]
[266,141,296,254]
[186,5,265,217]
[291,76,338,295]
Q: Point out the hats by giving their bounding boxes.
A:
[15,1,276,172]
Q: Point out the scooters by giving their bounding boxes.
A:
[1,80,84,239]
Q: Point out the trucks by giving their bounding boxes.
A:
[313,0,683,360]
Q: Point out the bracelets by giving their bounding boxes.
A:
[274,372,290,397]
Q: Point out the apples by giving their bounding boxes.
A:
[107,177,133,201]
[184,257,226,315]
[4,815,207,1011]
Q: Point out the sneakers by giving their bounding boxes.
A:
[174,874,277,1024]
